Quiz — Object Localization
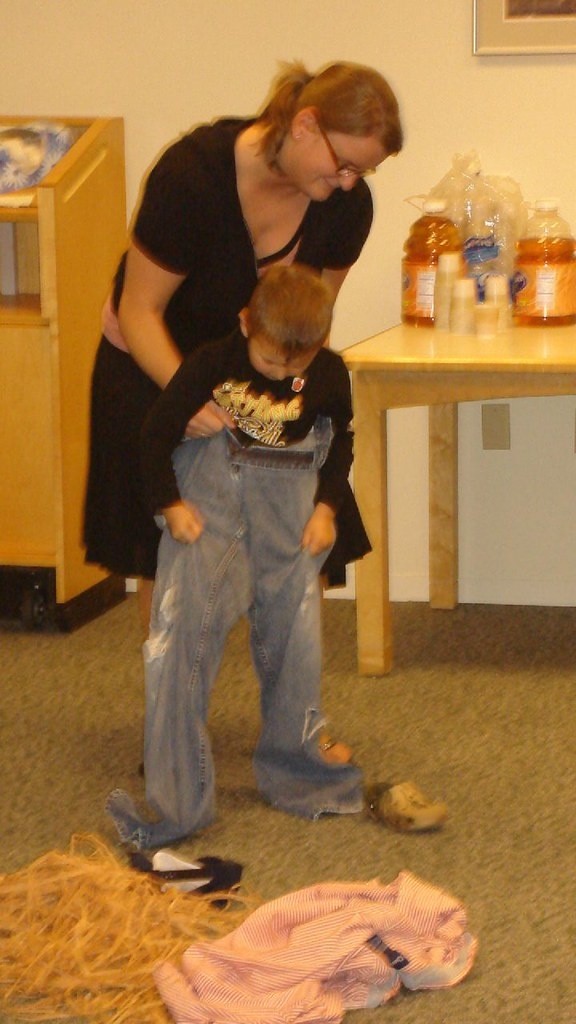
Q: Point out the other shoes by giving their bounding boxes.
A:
[317,732,355,767]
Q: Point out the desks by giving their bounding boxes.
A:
[339,321,576,678]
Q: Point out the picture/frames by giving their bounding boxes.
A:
[473,1,576,55]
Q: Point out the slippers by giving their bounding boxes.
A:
[367,779,448,833]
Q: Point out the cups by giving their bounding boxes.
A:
[484,274,510,329]
[473,305,497,341]
[449,278,479,334]
[435,251,465,330]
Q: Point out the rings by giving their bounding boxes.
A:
[201,432,207,438]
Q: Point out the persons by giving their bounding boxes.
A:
[80,61,404,852]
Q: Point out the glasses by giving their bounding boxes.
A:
[313,114,377,179]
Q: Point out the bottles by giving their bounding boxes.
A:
[401,194,467,328]
[514,200,576,326]
[432,149,510,300]
